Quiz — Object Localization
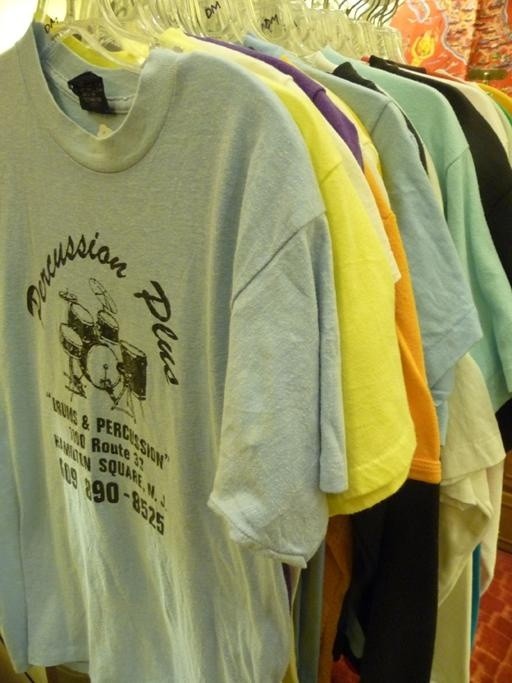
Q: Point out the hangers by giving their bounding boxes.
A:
[31,1,412,73]
